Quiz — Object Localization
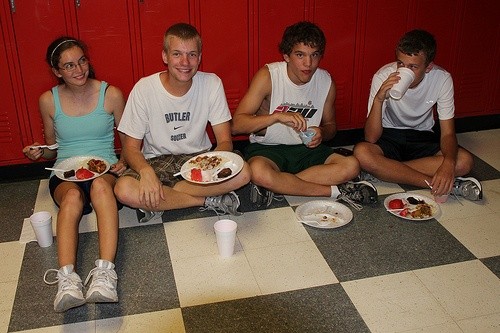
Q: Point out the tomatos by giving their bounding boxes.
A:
[400,208,409,216]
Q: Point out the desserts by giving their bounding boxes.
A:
[299,130,317,144]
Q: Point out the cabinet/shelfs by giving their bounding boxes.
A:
[0,0,500,167]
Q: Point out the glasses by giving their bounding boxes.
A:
[59,55,89,72]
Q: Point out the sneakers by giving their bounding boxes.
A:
[357,168,382,183]
[82,259,118,303]
[136,208,164,223]
[449,176,483,206]
[335,180,378,211]
[43,264,84,312]
[198,190,243,219]
[246,180,284,207]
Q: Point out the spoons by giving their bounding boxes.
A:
[213,161,233,177]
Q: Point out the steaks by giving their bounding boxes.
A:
[64,169,75,178]
[217,167,232,178]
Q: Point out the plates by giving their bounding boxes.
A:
[180,150,244,184]
[54,155,110,182]
[383,192,440,221]
[295,199,353,229]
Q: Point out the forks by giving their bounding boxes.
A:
[30,143,59,150]
[297,220,330,227]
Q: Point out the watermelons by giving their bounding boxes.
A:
[76,167,96,180]
[389,199,403,208]
[190,168,202,181]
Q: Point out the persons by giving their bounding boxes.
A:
[23,36,128,312]
[353,30,483,205]
[113,23,254,223]
[230,21,380,211]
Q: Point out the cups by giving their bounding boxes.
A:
[214,219,238,257]
[298,128,317,146]
[388,67,415,100]
[30,211,53,248]
